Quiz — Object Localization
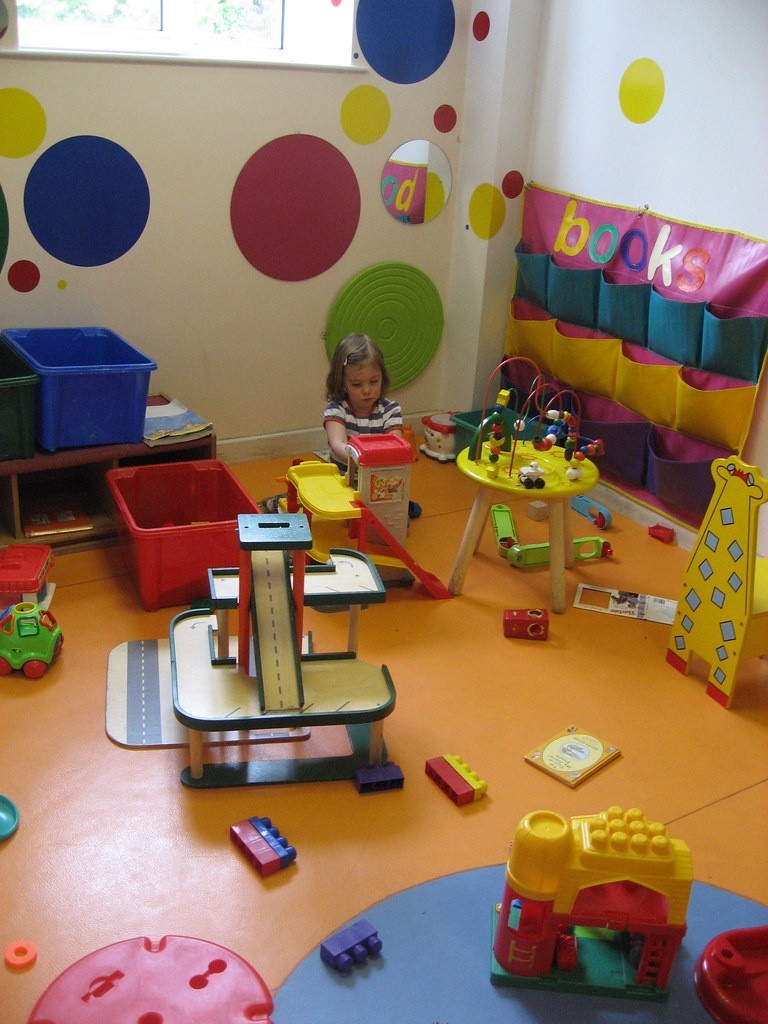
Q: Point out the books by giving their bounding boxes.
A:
[21,499,94,539]
[144,399,215,447]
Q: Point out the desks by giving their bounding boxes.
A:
[448,439,599,613]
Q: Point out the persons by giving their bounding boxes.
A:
[323,332,422,518]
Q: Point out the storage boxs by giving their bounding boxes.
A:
[105,459,264,612]
[0,335,38,461]
[0,327,157,453]
[450,405,566,465]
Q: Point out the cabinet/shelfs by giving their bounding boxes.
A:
[1,393,217,556]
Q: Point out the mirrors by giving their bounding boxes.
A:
[380,139,454,225]
